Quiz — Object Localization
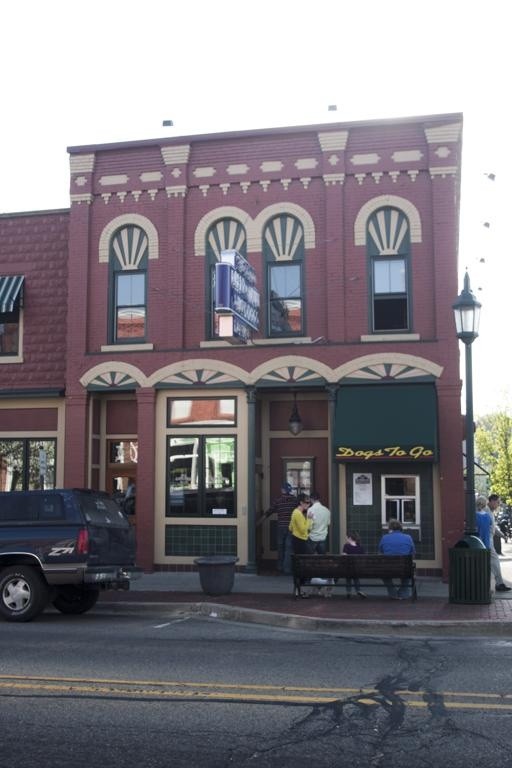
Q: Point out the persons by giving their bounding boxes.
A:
[289,493,316,600]
[255,482,295,572]
[485,494,511,594]
[340,529,368,601]
[476,495,492,558]
[306,490,334,590]
[377,517,415,600]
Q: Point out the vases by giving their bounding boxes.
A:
[193,557,238,597]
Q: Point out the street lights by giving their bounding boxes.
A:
[448,270,492,605]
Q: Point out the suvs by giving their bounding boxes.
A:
[0,488,142,623]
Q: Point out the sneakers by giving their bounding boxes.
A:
[496,584,511,591]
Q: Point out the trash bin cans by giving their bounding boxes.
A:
[450,536,490,604]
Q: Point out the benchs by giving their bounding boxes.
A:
[290,556,420,603]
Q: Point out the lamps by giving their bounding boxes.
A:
[287,390,305,439]
